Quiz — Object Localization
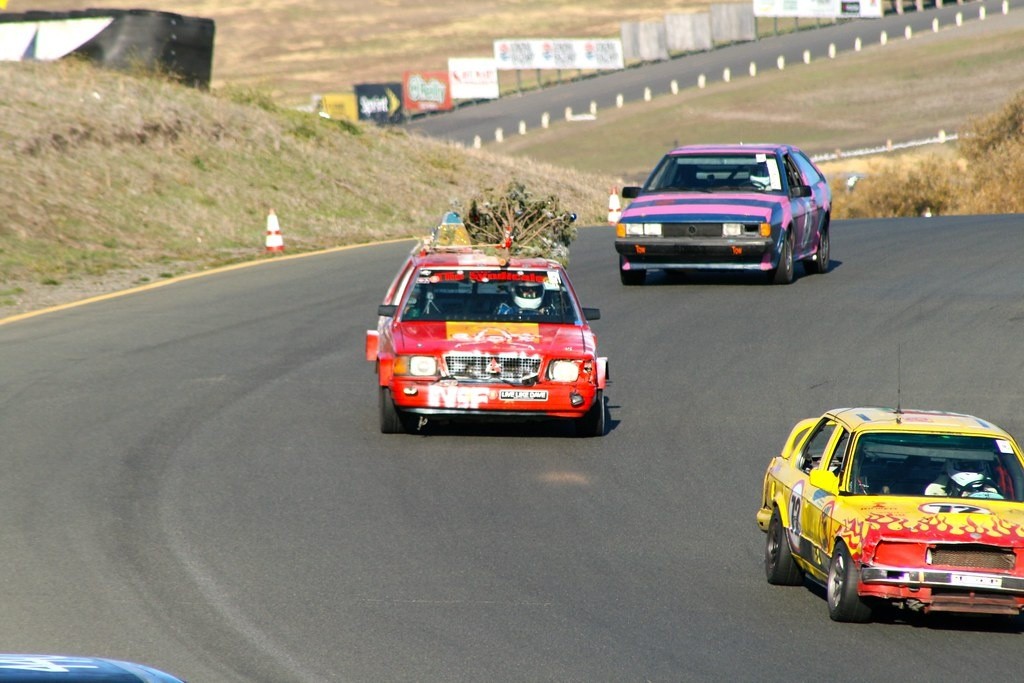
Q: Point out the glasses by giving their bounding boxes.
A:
[522,286,538,292]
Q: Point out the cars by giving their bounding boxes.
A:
[615,142,833,287]
[366,211,609,438]
[756,406,1024,631]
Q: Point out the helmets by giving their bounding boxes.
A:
[945,459,986,488]
[511,282,545,308]
[750,162,770,186]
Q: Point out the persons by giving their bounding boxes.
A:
[497,283,558,316]
[925,457,996,498]
[746,167,772,191]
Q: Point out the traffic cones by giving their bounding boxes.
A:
[264,208,286,258]
[606,184,623,226]
